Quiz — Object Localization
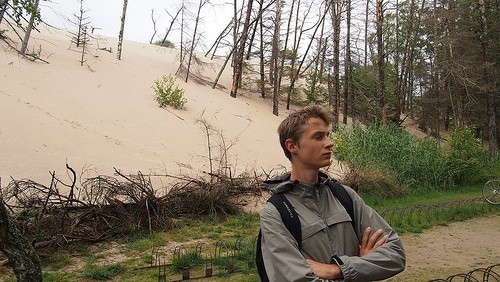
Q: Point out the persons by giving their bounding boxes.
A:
[259,106,407,282]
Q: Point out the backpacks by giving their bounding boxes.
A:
[255,178,354,282]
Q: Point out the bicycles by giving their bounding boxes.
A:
[483,178,500,205]
